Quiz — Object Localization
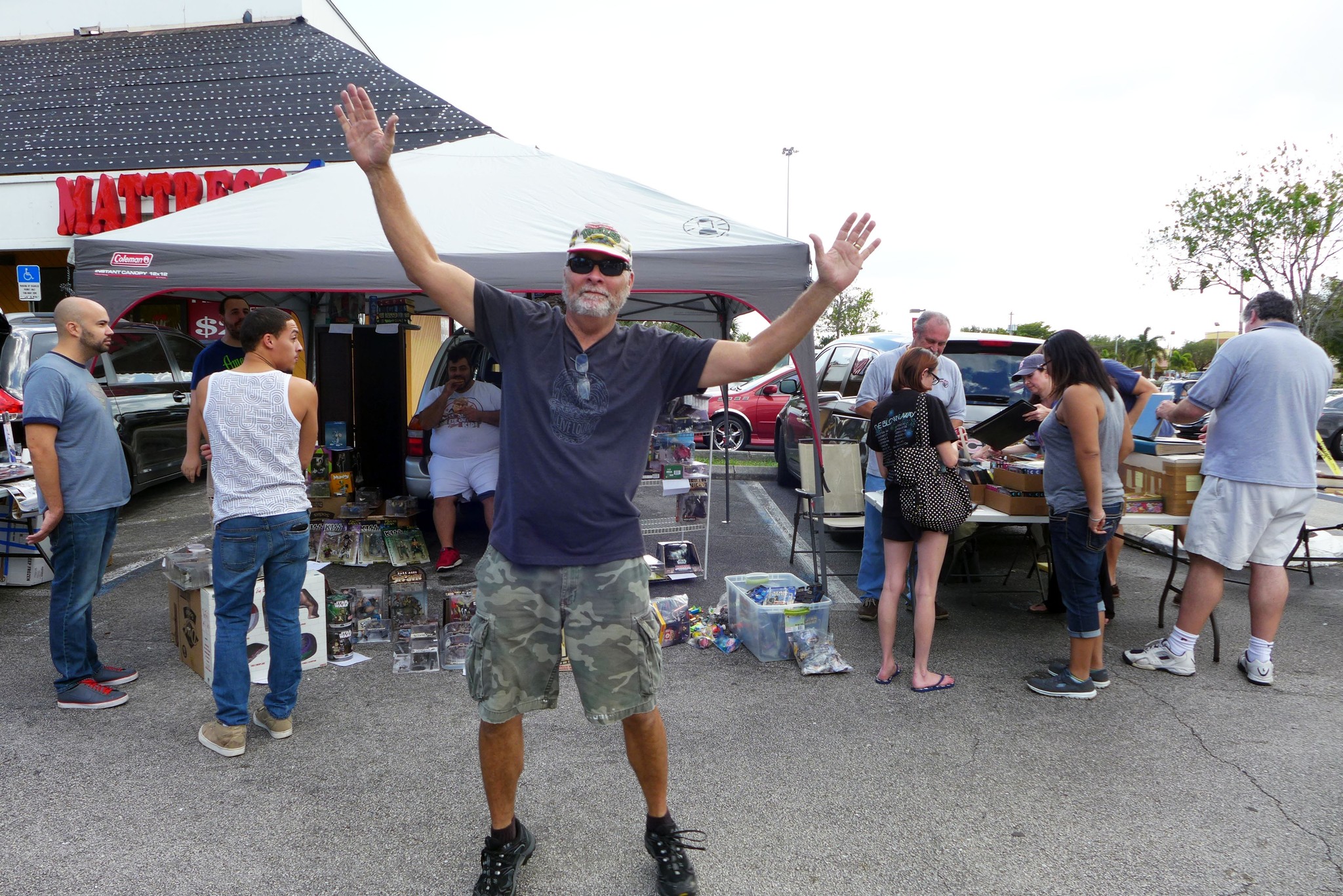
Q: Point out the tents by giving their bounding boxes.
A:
[74,130,829,632]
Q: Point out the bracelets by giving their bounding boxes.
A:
[1090,508,1105,522]
[1000,450,1003,454]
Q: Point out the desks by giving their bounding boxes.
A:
[864,490,1220,661]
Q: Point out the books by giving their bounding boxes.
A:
[1131,392,1206,457]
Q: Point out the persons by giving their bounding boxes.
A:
[196,308,318,756]
[409,347,500,572]
[1123,290,1334,685]
[21,294,137,710]
[988,354,1116,625]
[1026,329,1135,701]
[1031,343,1160,598]
[328,84,881,894]
[855,311,967,622]
[181,295,252,536]
[865,347,961,694]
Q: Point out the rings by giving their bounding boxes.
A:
[855,244,860,249]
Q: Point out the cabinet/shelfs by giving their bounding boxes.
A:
[626,425,714,583]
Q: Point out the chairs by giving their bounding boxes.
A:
[788,439,867,582]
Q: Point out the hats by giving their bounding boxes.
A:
[566,222,633,267]
[1011,353,1046,381]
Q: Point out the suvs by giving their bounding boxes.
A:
[404,326,501,500]
[774,332,1047,488]
[0,311,208,512]
[699,364,800,450]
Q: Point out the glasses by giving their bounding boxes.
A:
[566,256,631,276]
[569,353,591,401]
[926,371,940,386]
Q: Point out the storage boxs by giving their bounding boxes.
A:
[656,541,702,574]
[162,544,327,692]
[957,454,1204,516]
[0,497,56,587]
[643,554,664,580]
[659,460,709,477]
[723,572,831,663]
[355,583,476,675]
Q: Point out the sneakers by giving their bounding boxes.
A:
[906,600,948,619]
[198,718,247,757]
[1171,588,1188,607]
[57,679,129,709]
[1112,583,1121,597]
[1047,662,1110,689]
[858,597,880,621]
[472,816,537,896]
[644,822,699,896]
[436,547,463,571]
[252,703,293,739]
[1122,638,1197,676]
[1025,666,1098,700]
[87,664,138,686]
[1236,648,1275,686]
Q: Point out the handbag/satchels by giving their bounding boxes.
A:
[894,392,974,531]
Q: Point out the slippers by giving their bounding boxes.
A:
[875,662,901,684]
[1029,600,1065,616]
[911,672,956,692]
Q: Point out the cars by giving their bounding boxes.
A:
[1157,370,1343,462]
